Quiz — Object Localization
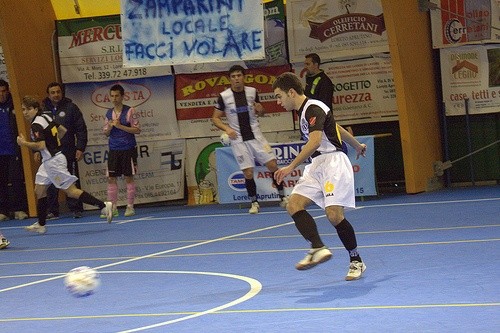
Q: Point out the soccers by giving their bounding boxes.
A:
[64,265,102,299]
[220,132,232,147]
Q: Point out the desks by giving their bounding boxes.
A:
[215,134,391,209]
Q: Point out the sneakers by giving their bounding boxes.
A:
[280,197,289,208]
[72,212,82,219]
[46,213,59,220]
[296,247,332,270]
[100,210,119,218]
[25,222,46,232]
[249,202,260,214]
[101,202,113,223]
[124,206,135,216]
[345,257,366,280]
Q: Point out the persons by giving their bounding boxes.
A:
[16,95,113,233]
[100,85,142,219]
[272,72,366,281]
[304,53,334,112]
[211,65,289,213]
[30,82,88,221]
[0,79,28,222]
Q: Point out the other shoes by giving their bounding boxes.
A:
[0,238,10,249]
